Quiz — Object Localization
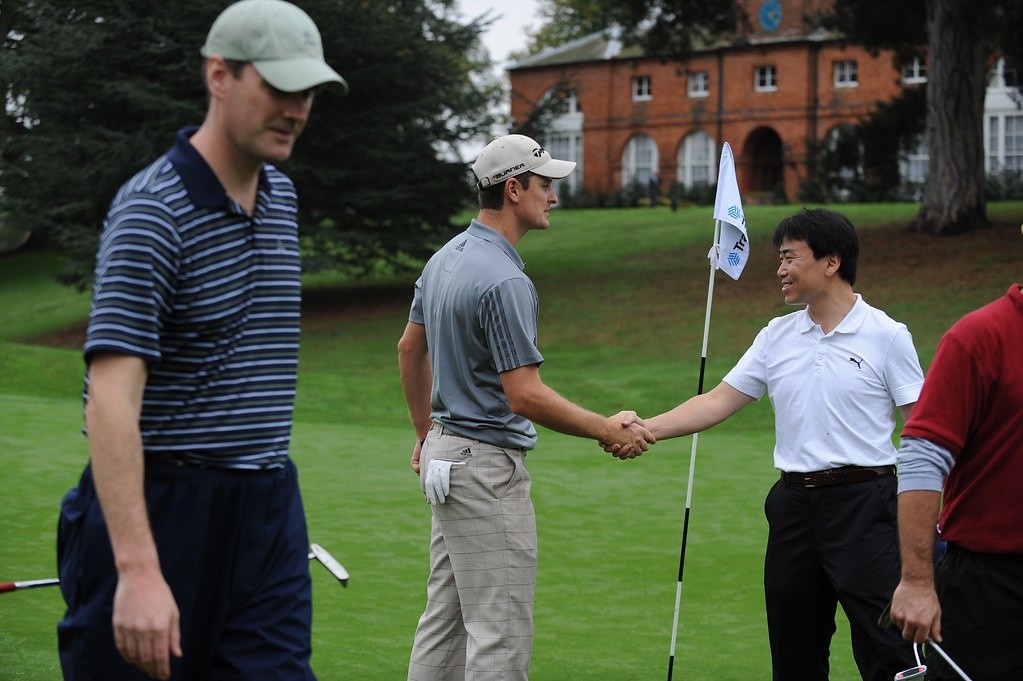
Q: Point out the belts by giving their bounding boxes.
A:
[780,464,897,489]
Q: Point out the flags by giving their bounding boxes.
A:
[713,142,753,282]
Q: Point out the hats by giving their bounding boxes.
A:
[200,0,352,96]
[470,134,579,190]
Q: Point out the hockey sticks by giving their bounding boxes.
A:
[0,541,350,595]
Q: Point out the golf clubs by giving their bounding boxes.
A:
[877,602,973,681]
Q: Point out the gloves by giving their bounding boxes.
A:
[426,457,452,507]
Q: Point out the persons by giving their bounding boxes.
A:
[56,0,317,681]
[599,207,925,681]
[397,134,656,681]
[891,282,1023,680]
